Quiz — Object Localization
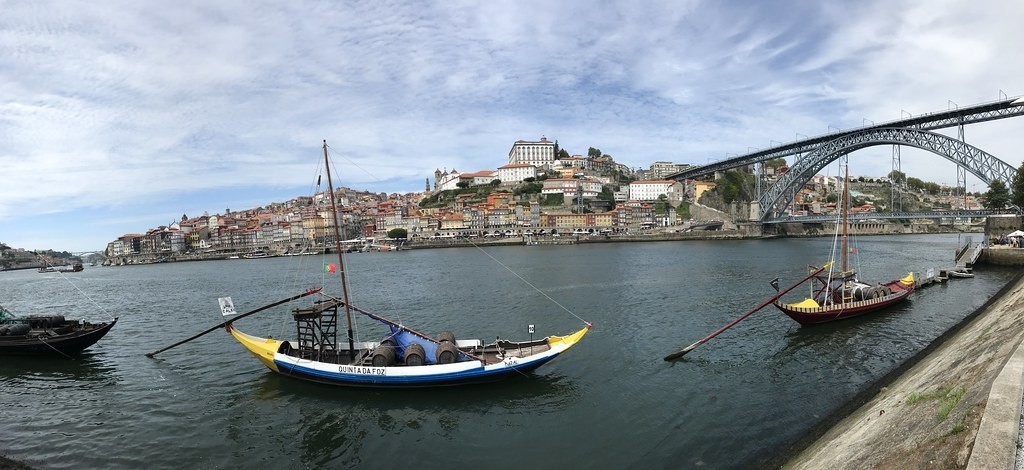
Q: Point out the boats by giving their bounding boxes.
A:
[37,261,84,272]
[88,259,126,267]
[975,207,1024,268]
[0,306,119,358]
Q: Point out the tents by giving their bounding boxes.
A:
[1007,230,1024,247]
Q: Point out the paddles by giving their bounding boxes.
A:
[664,260,835,360]
[144,285,323,356]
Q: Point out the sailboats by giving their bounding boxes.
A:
[220,138,594,390]
[769,155,922,326]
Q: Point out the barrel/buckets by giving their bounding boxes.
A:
[438,331,455,345]
[854,285,892,299]
[404,344,425,366]
[372,344,396,367]
[435,341,457,364]
[0,314,64,335]
[381,335,396,346]
